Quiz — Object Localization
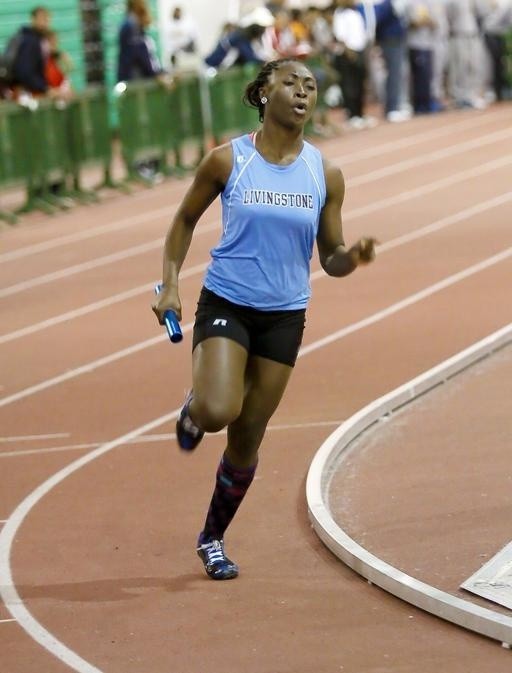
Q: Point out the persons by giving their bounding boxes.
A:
[153,57,382,580]
[171,1,511,131]
[0,6,75,194]
[117,1,165,181]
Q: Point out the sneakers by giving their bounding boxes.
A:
[198,535,236,581]
[176,389,205,452]
